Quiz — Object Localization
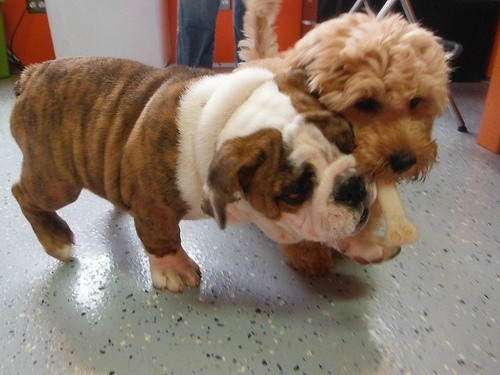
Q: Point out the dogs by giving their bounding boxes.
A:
[7,56,378,295]
[236,0,460,265]
